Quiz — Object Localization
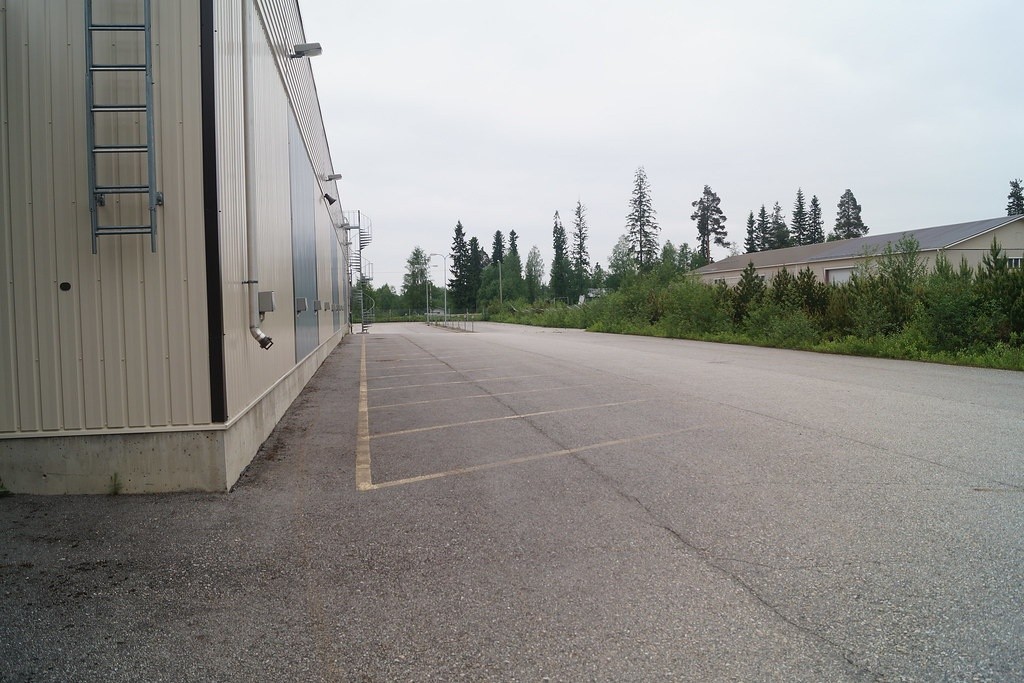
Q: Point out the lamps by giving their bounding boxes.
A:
[339,223,350,228]
[326,174,342,181]
[341,242,352,246]
[324,193,337,205]
[290,43,323,58]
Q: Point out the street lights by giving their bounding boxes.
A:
[431,253,460,327]
[497,260,502,303]
[415,265,438,326]
